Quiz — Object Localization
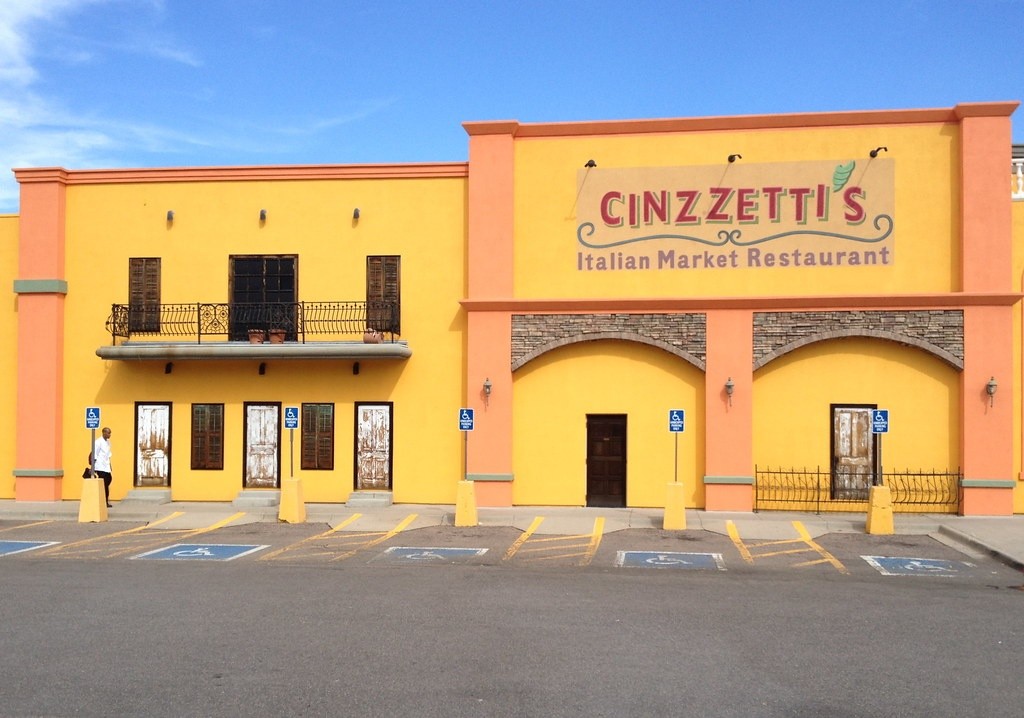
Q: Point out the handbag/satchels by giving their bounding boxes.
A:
[83,468,96,478]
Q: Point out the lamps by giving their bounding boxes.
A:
[258,363,265,375]
[986,377,997,407]
[165,362,173,373]
[483,378,492,408]
[167,209,174,221]
[726,377,734,407]
[260,209,267,219]
[354,209,360,218]
[585,159,597,168]
[353,362,360,376]
[870,147,888,157]
[728,154,742,162]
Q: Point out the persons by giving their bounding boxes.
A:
[89,427,113,507]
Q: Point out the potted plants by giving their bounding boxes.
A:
[248,314,287,344]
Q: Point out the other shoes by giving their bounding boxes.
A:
[107,502,112,507]
